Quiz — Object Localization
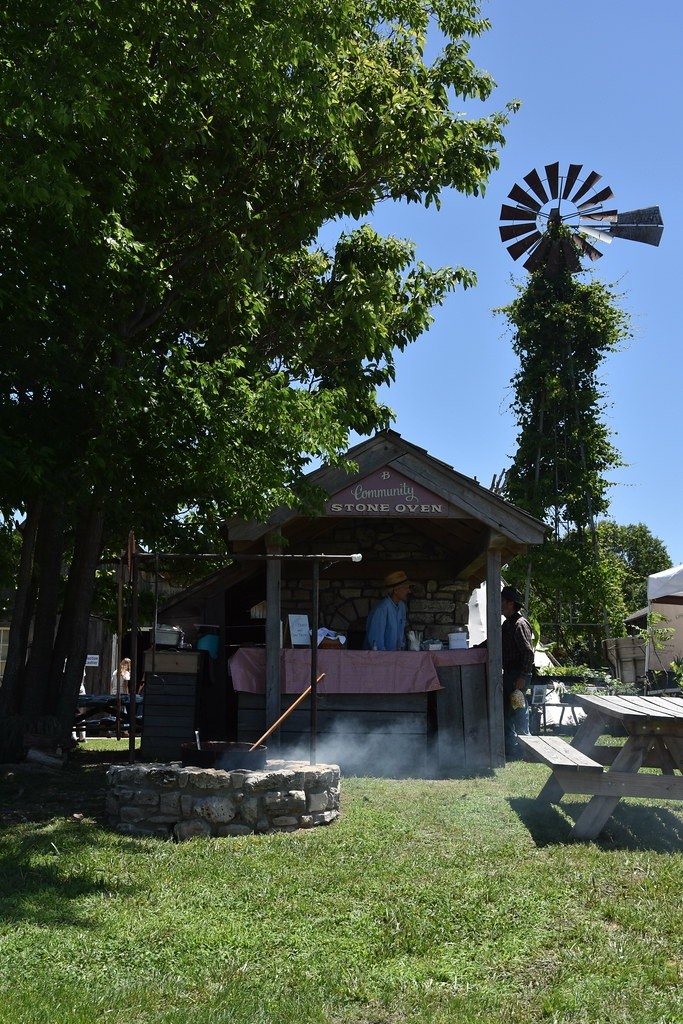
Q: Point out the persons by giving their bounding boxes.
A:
[70,663,87,742]
[357,570,415,651]
[108,657,131,738]
[473,586,533,761]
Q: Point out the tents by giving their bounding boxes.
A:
[643,563,683,697]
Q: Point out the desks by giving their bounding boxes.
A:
[530,694,683,844]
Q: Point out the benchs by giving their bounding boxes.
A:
[516,734,606,773]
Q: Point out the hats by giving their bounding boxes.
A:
[501,586,527,612]
[381,570,417,597]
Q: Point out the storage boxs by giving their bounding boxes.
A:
[142,650,202,673]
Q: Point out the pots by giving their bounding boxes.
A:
[179,741,267,771]
